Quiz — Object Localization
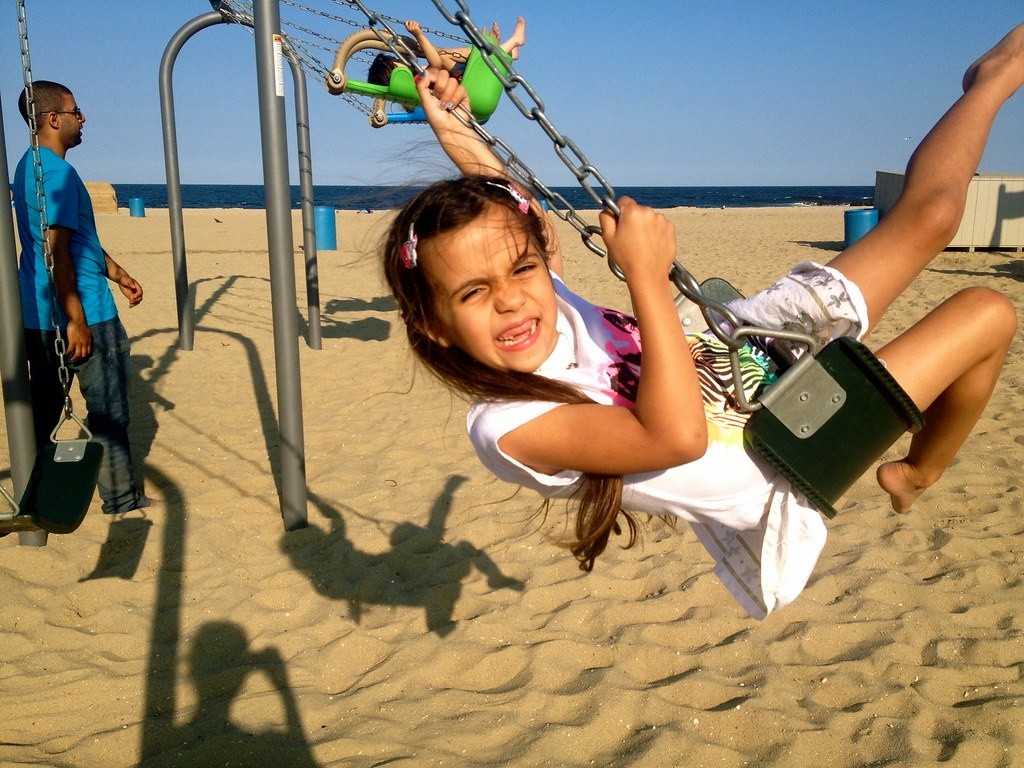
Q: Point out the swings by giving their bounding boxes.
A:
[1,0,104,535]
[352,0,925,520]
[222,1,512,119]
[223,1,491,125]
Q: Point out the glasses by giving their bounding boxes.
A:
[41,107,82,120]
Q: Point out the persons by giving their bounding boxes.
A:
[367,17,526,88]
[384,20,1024,623]
[13,81,168,516]
